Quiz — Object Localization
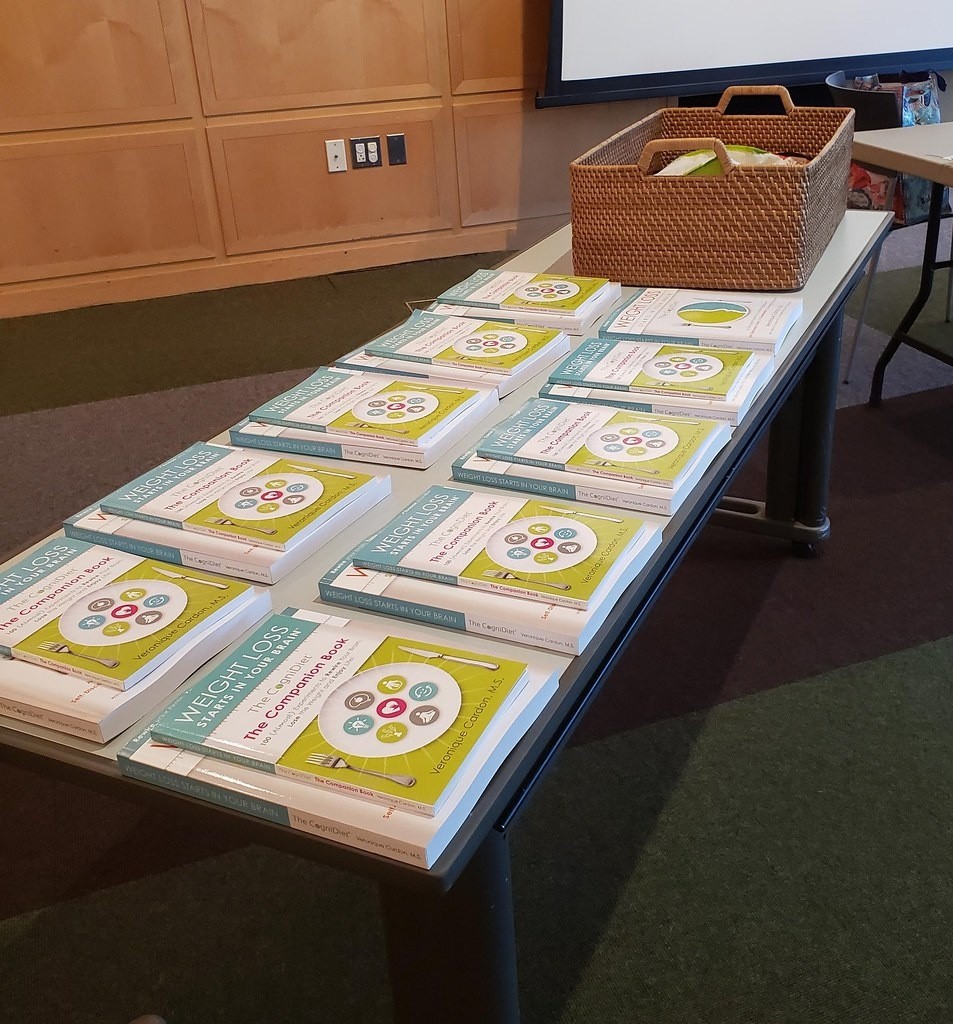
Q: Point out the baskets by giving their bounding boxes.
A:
[568,86,855,292]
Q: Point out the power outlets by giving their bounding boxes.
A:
[349,135,383,169]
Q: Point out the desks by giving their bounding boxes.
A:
[0,209,895,1024]
[852,121,953,412]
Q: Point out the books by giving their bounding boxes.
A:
[64,269,804,657]
[0,536,559,870]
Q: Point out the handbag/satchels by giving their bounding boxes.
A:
[847,68,953,227]
